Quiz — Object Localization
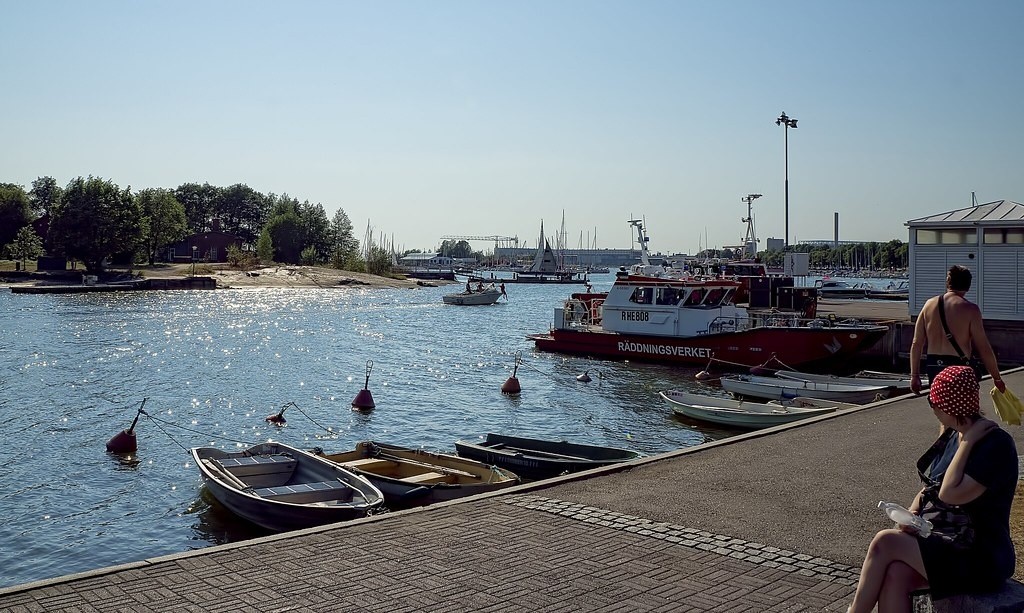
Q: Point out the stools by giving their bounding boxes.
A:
[909,575,1024,613]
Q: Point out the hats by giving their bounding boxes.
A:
[930,363,980,416]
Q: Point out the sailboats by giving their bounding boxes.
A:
[455,208,610,275]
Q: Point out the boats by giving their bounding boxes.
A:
[816,276,872,299]
[767,396,861,412]
[409,262,455,280]
[468,268,591,284]
[308,439,522,507]
[719,370,929,405]
[455,432,648,480]
[191,441,385,532]
[443,282,503,305]
[867,279,909,300]
[659,389,839,429]
[525,193,889,374]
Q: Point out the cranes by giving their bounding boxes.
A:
[440,234,519,248]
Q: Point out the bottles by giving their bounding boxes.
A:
[878,501,933,538]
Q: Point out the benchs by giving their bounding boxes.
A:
[501,447,519,453]
[399,472,456,484]
[207,454,297,489]
[244,480,353,504]
[557,455,568,461]
[173,256,192,264]
[338,457,399,471]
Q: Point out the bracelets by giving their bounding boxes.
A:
[994,380,1003,382]
[910,373,919,376]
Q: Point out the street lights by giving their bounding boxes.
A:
[192,245,197,278]
[775,110,799,251]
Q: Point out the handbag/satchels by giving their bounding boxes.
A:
[916,482,974,553]
[968,352,980,381]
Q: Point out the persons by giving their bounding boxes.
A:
[500,283,508,301]
[466,280,475,294]
[478,281,484,293]
[910,264,1006,438]
[845,366,1018,612]
[540,274,543,280]
